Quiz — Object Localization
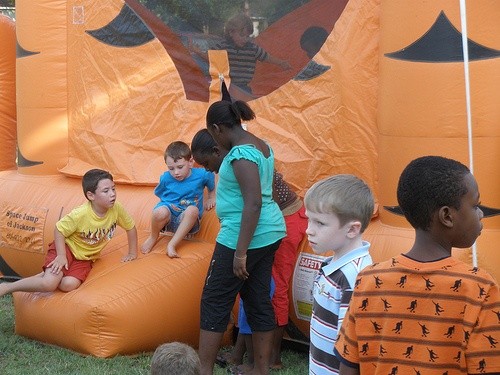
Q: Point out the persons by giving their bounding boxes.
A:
[141,141,215,258]
[303,174,374,375]
[333,156,500,375]
[0,169,138,295]
[191,100,308,375]
[194,14,292,94]
[151,341,201,375]
[300,27,329,61]
